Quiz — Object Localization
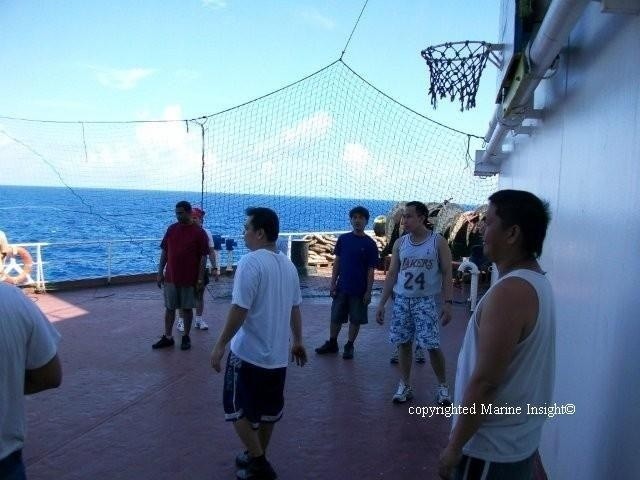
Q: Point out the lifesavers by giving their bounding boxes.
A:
[1,246,32,284]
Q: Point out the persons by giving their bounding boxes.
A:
[437,189,558,479]
[0,281,63,480]
[209,205,308,480]
[314,205,377,361]
[177,207,219,334]
[151,200,208,354]
[388,346,428,363]
[370,202,455,408]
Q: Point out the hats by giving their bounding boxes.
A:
[191,208,203,218]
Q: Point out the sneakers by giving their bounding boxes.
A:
[152,334,175,349]
[181,334,190,350]
[438,384,451,406]
[315,341,427,364]
[194,320,208,330]
[236,450,250,467]
[392,385,414,402]
[236,468,280,480]
[177,320,184,332]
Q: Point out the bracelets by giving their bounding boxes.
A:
[444,297,454,304]
[211,267,218,271]
[197,279,204,284]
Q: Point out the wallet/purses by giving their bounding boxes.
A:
[421,41,491,112]
